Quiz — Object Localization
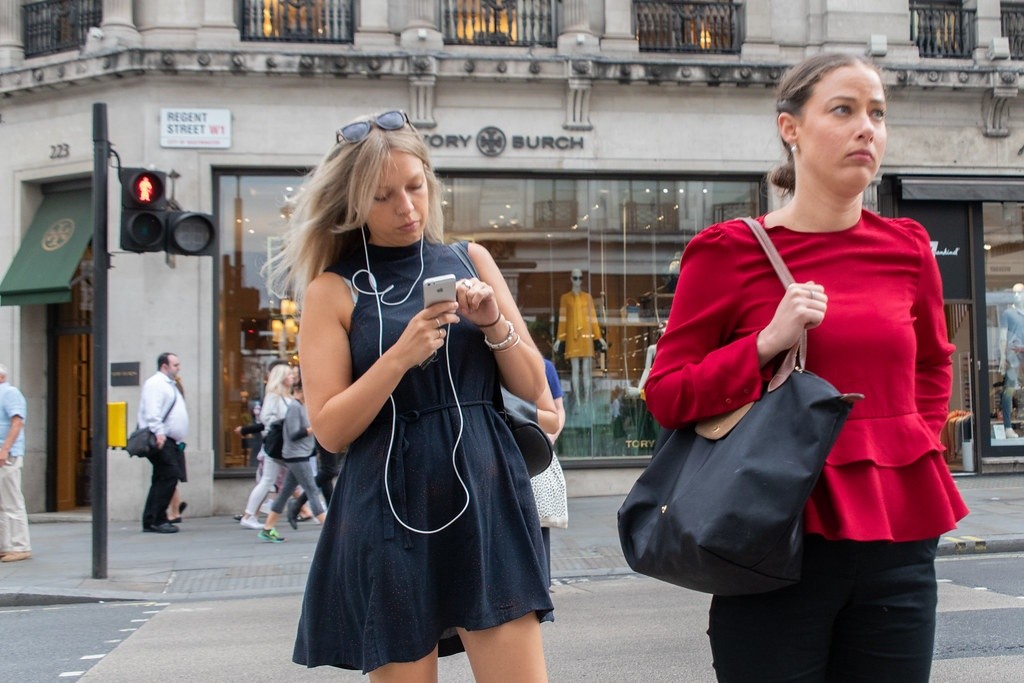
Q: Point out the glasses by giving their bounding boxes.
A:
[573,276,582,280]
[336,109,411,144]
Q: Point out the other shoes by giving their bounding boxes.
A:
[169,517,181,523]
[240,516,266,530]
[234,514,243,520]
[179,502,186,513]
[0,552,32,561]
[288,499,297,529]
[297,516,311,521]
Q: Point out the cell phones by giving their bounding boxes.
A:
[423,274,456,313]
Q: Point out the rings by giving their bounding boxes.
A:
[435,318,441,328]
[462,280,473,288]
[810,290,814,299]
[438,329,442,338]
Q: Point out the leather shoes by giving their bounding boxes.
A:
[144,523,178,533]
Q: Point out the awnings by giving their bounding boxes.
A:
[0,188,92,306]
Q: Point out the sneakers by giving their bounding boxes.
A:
[257,527,287,542]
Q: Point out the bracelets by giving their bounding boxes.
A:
[473,311,501,328]
[490,334,521,352]
[485,321,515,349]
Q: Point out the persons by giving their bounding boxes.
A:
[998,284,1024,438]
[137,352,189,533]
[637,320,669,438]
[233,360,348,543]
[295,110,566,683]
[644,54,971,683]
[553,266,608,415]
[0,364,32,562]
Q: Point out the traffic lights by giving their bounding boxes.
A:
[118,165,172,252]
[164,211,219,259]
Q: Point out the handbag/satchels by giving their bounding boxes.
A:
[530,451,568,529]
[617,217,865,599]
[126,427,150,457]
[262,418,317,458]
[448,241,553,479]
